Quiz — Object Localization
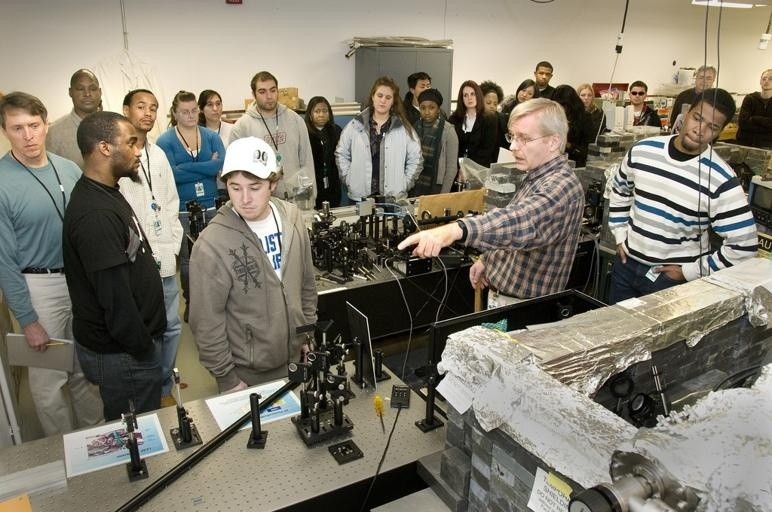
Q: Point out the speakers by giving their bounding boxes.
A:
[624,104,634,129]
[606,107,623,132]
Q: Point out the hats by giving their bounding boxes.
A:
[417,90,442,106]
[219,136,282,180]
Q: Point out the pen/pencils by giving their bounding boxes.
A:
[46,343,69,346]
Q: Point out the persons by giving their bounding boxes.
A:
[302,61,771,211]
[1,61,318,438]
[608,88,759,307]
[399,96,585,310]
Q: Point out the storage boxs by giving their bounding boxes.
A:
[244,87,300,112]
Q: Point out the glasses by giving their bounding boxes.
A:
[173,108,199,116]
[630,90,646,96]
[504,132,559,145]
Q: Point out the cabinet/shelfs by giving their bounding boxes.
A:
[354,46,453,119]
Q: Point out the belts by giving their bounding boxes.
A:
[20,267,65,273]
[488,284,534,299]
